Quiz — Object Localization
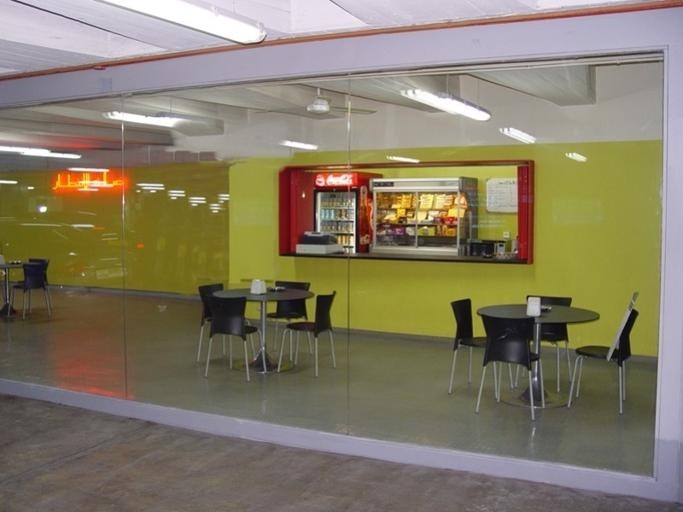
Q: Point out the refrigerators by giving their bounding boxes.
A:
[311,170,384,253]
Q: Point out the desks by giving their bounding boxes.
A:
[1,261,24,316]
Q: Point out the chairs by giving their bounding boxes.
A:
[195,278,340,383]
[7,257,55,320]
[446,292,640,422]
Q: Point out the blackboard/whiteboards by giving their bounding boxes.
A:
[486,178,518,214]
